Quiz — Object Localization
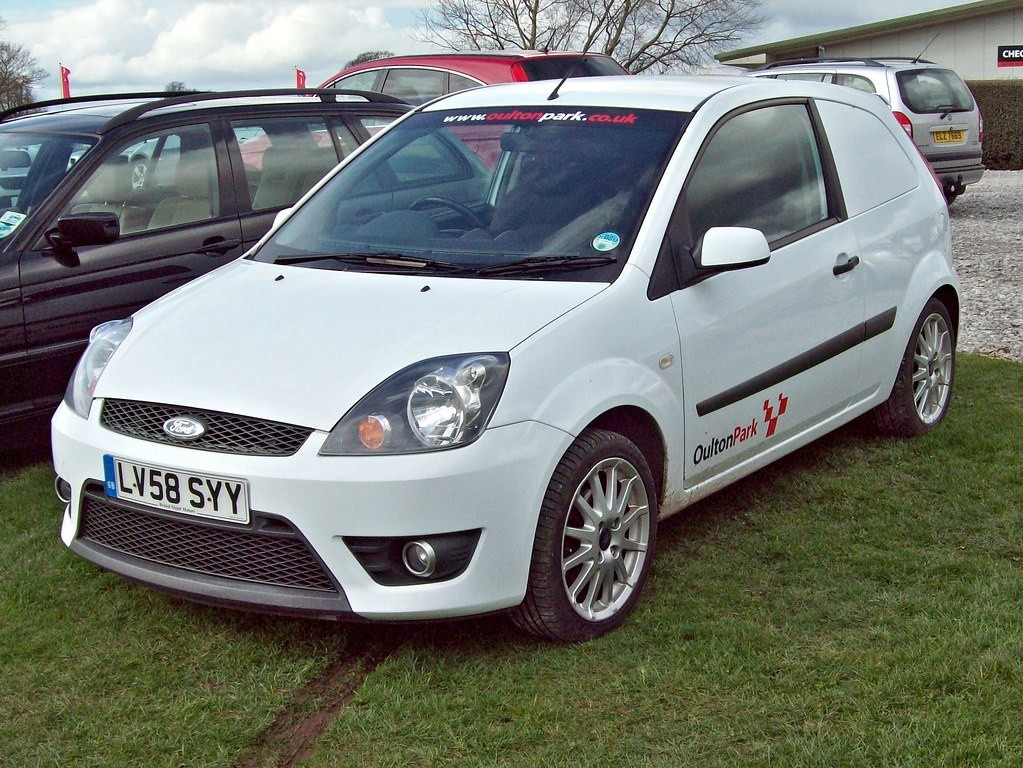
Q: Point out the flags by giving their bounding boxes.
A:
[296,69,306,88]
[62,67,70,98]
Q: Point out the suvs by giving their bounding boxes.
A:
[0,52,629,466]
[743,56,986,207]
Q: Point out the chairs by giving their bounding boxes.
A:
[489,153,576,236]
[72,155,146,234]
[147,150,212,229]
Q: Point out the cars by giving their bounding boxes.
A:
[52,78,959,640]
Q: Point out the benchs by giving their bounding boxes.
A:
[253,147,440,210]
